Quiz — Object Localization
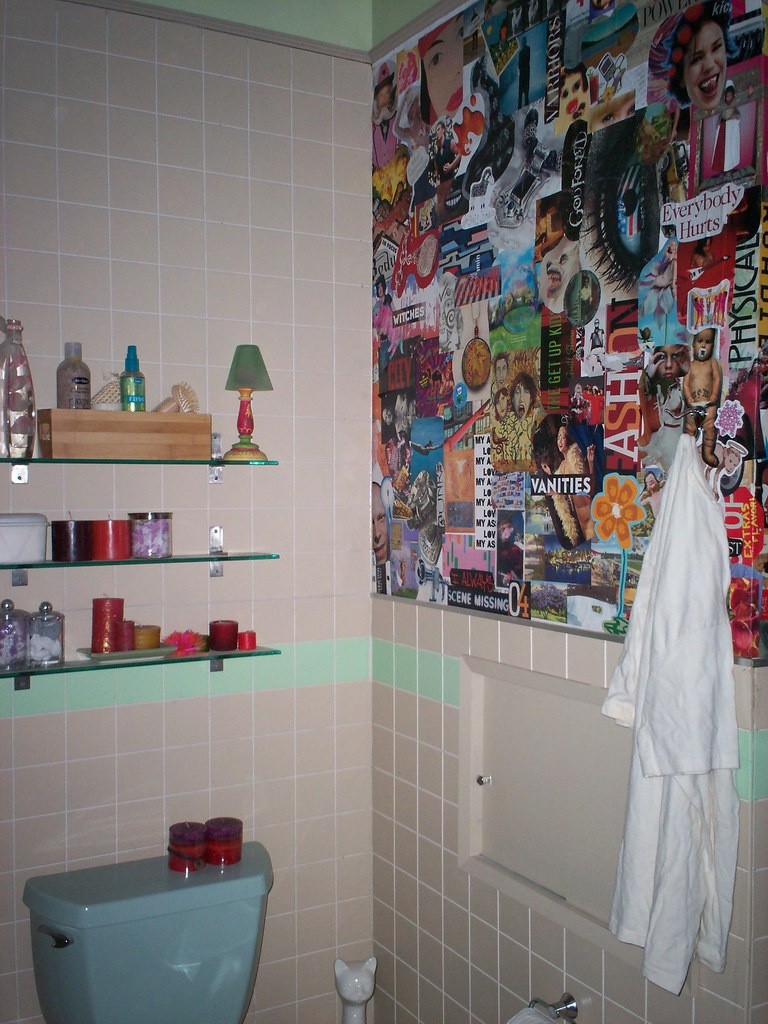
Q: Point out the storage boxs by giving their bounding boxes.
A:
[38,409,212,460]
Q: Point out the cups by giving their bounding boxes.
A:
[92,519,132,561]
[51,520,92,562]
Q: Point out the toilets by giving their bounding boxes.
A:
[23,841,275,1024]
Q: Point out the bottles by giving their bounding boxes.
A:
[120,345,146,412]
[0,319,37,458]
[56,342,91,410]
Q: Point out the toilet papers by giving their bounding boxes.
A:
[507,1007,560,1024]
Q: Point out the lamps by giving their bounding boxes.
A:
[221,344,274,461]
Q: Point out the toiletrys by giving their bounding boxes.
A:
[56,343,92,408]
[120,345,146,412]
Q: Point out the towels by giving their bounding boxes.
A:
[600,433,739,995]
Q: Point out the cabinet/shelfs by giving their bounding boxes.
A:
[0,455,281,692]
